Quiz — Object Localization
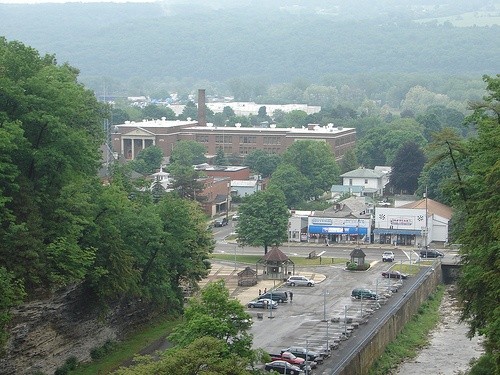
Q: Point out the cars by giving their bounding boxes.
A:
[263,360,302,375]
[246,298,278,309]
[280,346,319,362]
[381,270,408,279]
[231,213,240,221]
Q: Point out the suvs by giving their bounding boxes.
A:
[213,216,227,227]
[350,287,378,300]
[257,292,289,304]
[419,249,443,259]
[381,250,395,262]
[285,275,315,287]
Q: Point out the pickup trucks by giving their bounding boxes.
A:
[269,351,307,367]
